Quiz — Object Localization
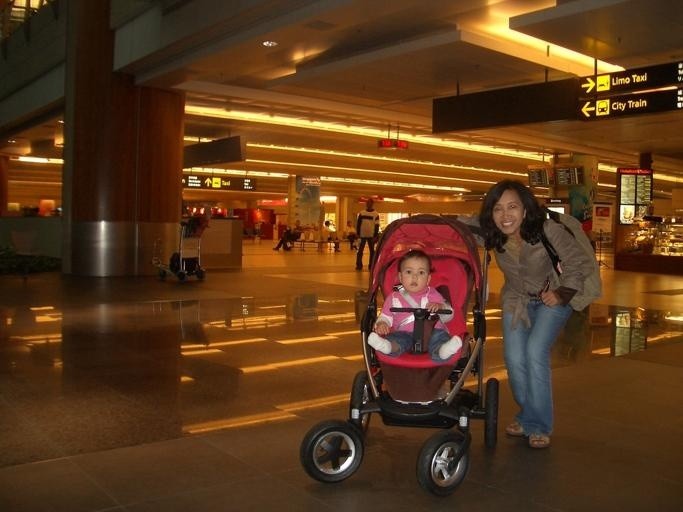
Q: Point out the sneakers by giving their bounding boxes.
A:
[356,265,362,269]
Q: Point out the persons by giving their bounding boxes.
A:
[461,179,595,450]
[322,220,342,252]
[344,220,358,249]
[366,249,465,365]
[355,197,380,270]
[272,228,301,251]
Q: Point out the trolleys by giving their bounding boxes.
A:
[154,211,209,284]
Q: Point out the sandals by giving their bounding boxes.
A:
[528,434,550,448]
[505,422,524,437]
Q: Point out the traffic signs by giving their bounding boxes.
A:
[184,175,257,191]
[578,60,683,96]
[575,89,681,119]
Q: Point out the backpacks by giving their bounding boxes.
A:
[539,204,603,314]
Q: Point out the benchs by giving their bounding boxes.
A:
[294,239,351,252]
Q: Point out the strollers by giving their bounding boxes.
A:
[299,213,500,499]
